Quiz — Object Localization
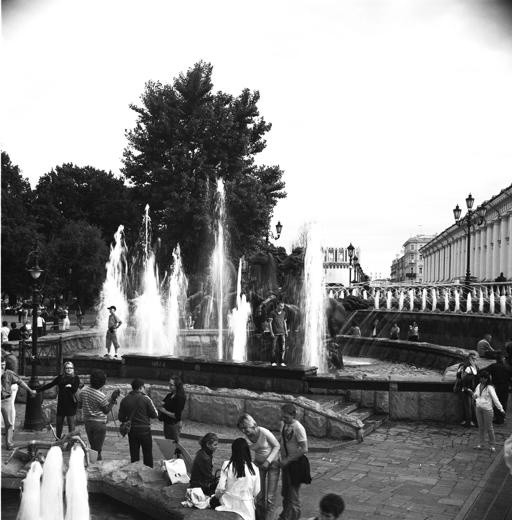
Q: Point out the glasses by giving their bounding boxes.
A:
[167,382,176,387]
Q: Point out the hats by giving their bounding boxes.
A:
[107,305,117,311]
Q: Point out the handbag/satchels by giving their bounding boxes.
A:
[72,388,82,405]
[213,469,222,499]
[118,419,132,438]
[287,454,313,488]
[160,456,194,486]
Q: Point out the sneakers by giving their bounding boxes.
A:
[474,442,496,453]
[460,420,475,427]
[100,353,112,359]
[112,354,119,360]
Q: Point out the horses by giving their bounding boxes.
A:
[239,286,304,362]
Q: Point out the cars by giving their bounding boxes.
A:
[445,275,482,295]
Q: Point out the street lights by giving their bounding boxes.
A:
[347,244,365,285]
[452,192,488,297]
[265,217,283,246]
[20,236,50,432]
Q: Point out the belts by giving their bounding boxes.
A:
[1,395,12,402]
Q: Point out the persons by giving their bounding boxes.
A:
[190,431,219,496]
[346,321,363,337]
[495,272,507,282]
[453,331,512,453]
[407,325,413,342]
[314,492,345,520]
[266,301,291,367]
[212,436,262,520]
[236,412,281,520]
[411,321,420,342]
[387,323,401,340]
[1,295,188,469]
[370,319,381,336]
[276,401,312,519]
